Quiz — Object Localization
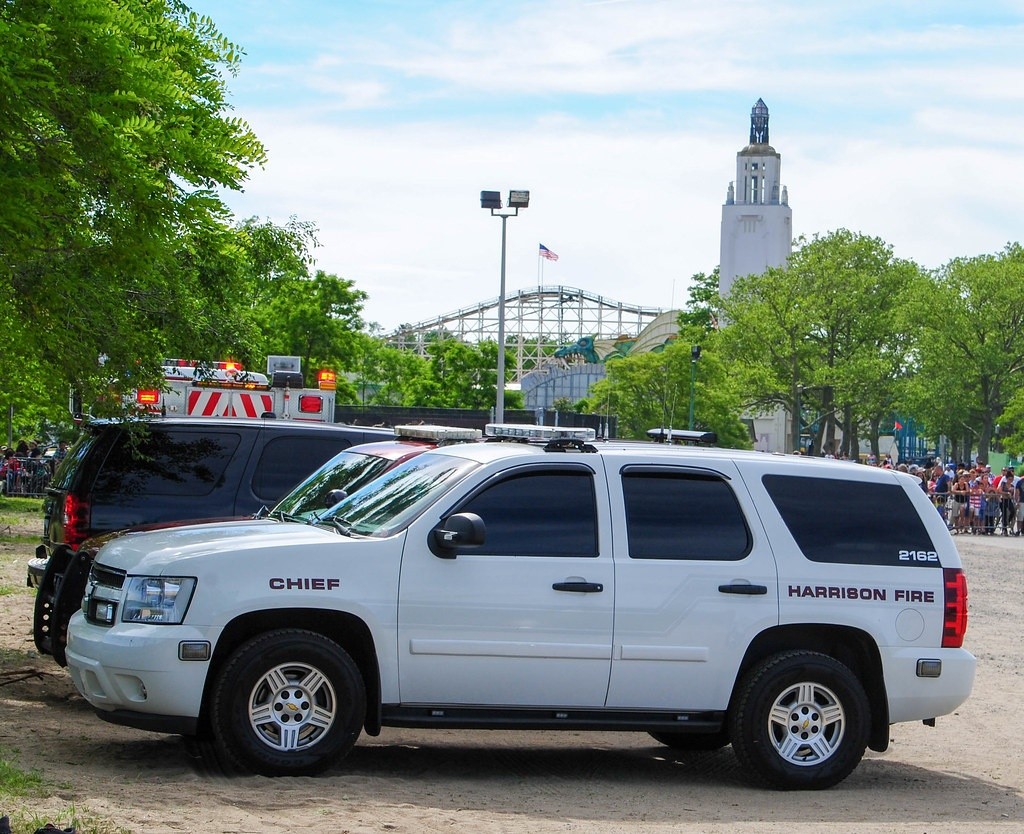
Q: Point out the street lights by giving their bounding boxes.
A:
[480,188,532,430]
[687,344,702,447]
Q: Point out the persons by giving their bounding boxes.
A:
[793,442,1024,538]
[0,438,68,498]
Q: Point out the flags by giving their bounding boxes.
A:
[539,244,558,261]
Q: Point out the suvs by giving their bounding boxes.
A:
[26,415,411,657]
[57,421,979,791]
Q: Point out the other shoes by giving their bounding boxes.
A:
[950,527,1024,537]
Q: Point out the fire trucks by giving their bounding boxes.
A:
[67,352,341,421]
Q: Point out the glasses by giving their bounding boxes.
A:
[1002,470,1008,471]
[1009,470,1014,472]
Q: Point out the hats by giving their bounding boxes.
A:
[963,471,969,475]
[1008,466,1015,469]
[917,467,926,473]
[985,465,991,468]
